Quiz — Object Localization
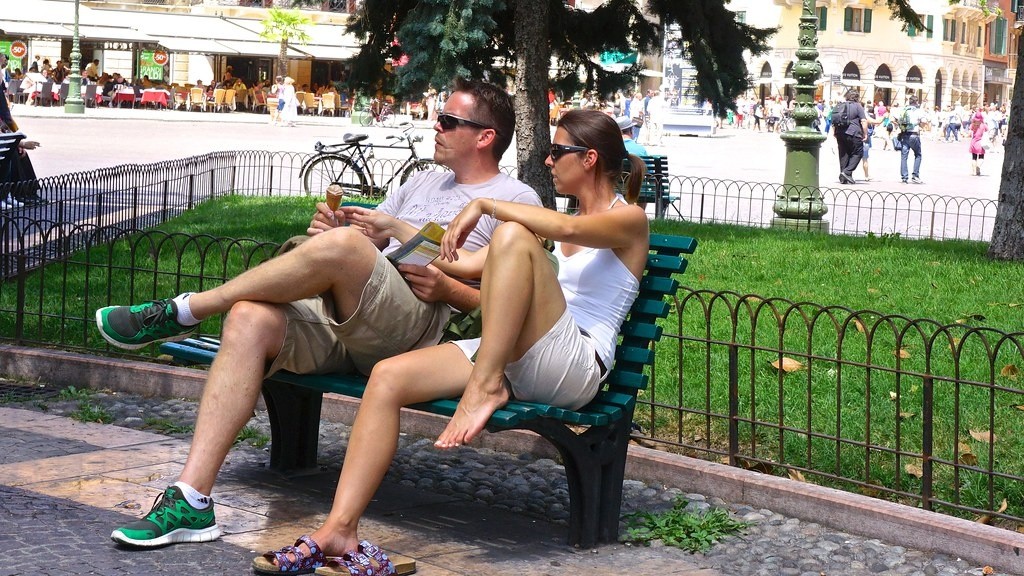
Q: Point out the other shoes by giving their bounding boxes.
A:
[840,172,855,184]
[16,196,38,206]
[902,179,908,184]
[841,179,847,184]
[1,201,12,210]
[32,193,49,204]
[912,176,925,183]
[7,192,24,207]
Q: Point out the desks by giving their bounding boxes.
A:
[82,86,103,103]
[32,85,58,107]
[141,89,168,108]
[113,87,135,108]
[181,91,211,112]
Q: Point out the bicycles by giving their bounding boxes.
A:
[297,119,452,201]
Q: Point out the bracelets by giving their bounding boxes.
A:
[490,197,496,218]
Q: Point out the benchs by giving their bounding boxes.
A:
[159,203,698,548]
[553,154,680,218]
[0,133,27,201]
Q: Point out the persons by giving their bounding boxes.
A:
[270,75,300,127]
[924,99,1008,153]
[252,109,649,576]
[865,100,902,150]
[716,95,796,132]
[834,89,888,184]
[898,94,928,184]
[301,82,352,118]
[816,99,836,134]
[194,65,272,103]
[423,82,452,121]
[0,53,49,210]
[969,106,989,175]
[96,81,544,546]
[616,115,655,210]
[14,55,151,107]
[550,88,671,146]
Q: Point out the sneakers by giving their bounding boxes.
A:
[96,298,198,350]
[110,485,221,546]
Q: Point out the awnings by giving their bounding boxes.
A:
[0,0,392,63]
[749,77,797,98]
[842,80,982,104]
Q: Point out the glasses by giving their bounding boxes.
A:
[437,112,504,137]
[549,143,590,160]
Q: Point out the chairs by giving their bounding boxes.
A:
[3,76,396,118]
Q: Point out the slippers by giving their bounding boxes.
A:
[315,540,416,576]
[253,534,344,575]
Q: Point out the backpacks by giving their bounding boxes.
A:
[831,102,858,129]
[900,106,918,130]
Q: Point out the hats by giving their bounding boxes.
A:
[615,116,637,130]
[973,113,984,121]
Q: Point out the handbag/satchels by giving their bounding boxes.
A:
[981,131,991,151]
[632,114,644,128]
[755,105,764,118]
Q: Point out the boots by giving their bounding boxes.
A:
[972,160,977,176]
[976,159,984,175]
